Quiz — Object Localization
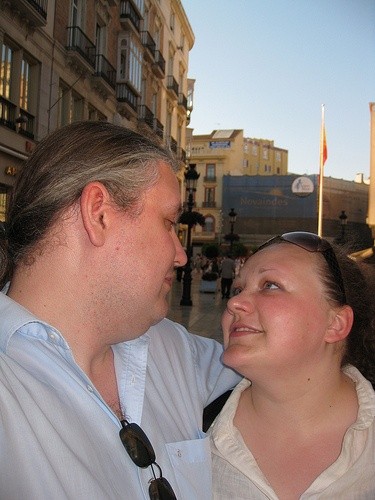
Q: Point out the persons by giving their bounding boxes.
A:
[205,231,375,500]
[220,253,235,300]
[0,118,245,500]
[191,246,245,279]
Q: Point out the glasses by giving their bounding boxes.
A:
[244,232,346,306]
[118,419,177,500]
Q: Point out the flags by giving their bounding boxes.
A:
[318,108,328,183]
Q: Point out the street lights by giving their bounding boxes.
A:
[339,209,348,245]
[224,208,241,250]
[179,164,201,306]
